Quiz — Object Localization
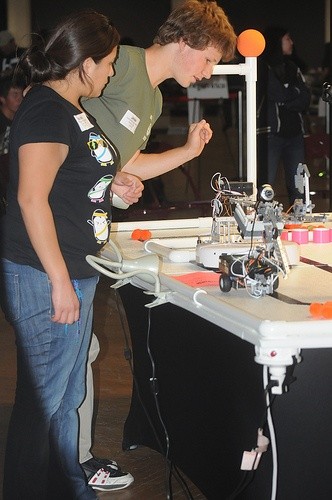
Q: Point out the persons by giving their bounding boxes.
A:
[254,28,313,213]
[0,9,28,213]
[0,7,145,500]
[19,0,239,492]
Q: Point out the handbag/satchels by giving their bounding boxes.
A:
[257,120,279,156]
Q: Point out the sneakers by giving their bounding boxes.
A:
[79,453,134,491]
